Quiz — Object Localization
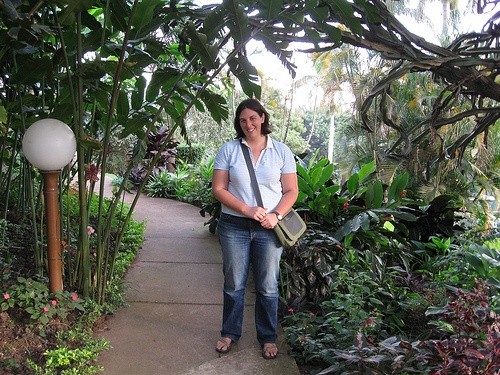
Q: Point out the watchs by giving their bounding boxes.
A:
[272,211,282,221]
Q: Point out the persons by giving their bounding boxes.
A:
[210,98,299,360]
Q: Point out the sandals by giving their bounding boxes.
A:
[216,337,232,353]
[262,342,279,360]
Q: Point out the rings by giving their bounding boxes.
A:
[271,226,274,229]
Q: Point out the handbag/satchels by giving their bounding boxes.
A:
[272,206,307,250]
